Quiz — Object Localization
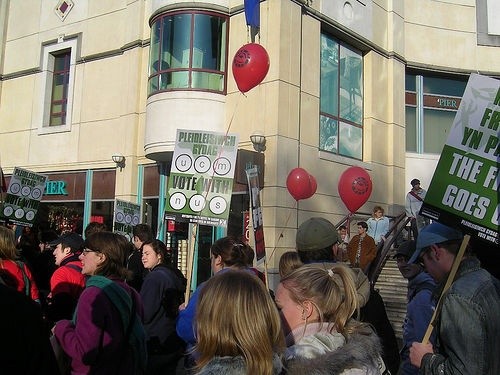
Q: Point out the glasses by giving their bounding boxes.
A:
[83,249,98,256]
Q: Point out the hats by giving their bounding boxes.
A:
[295,217,342,252]
[411,179,420,185]
[408,221,464,265]
[50,232,85,252]
[392,241,417,263]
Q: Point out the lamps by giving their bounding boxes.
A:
[250,134,266,153]
[112,155,125,172]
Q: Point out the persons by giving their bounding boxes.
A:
[365,206,390,246]
[0,220,187,375]
[176,216,399,375]
[348,222,377,271]
[393,222,500,375]
[337,225,351,265]
[405,179,431,241]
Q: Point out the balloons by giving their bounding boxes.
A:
[338,167,373,213]
[232,43,270,93]
[286,167,317,201]
